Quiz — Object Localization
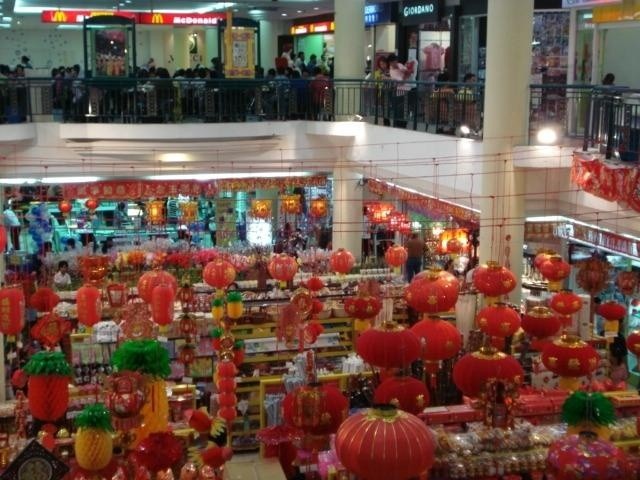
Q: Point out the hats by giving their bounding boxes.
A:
[386,53,401,62]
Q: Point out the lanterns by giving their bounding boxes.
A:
[1,175,640,480]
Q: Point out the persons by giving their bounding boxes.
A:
[51,64,86,122]
[100,57,224,122]
[375,52,410,127]
[1,64,28,122]
[433,75,485,133]
[600,73,622,97]
[252,44,334,121]
